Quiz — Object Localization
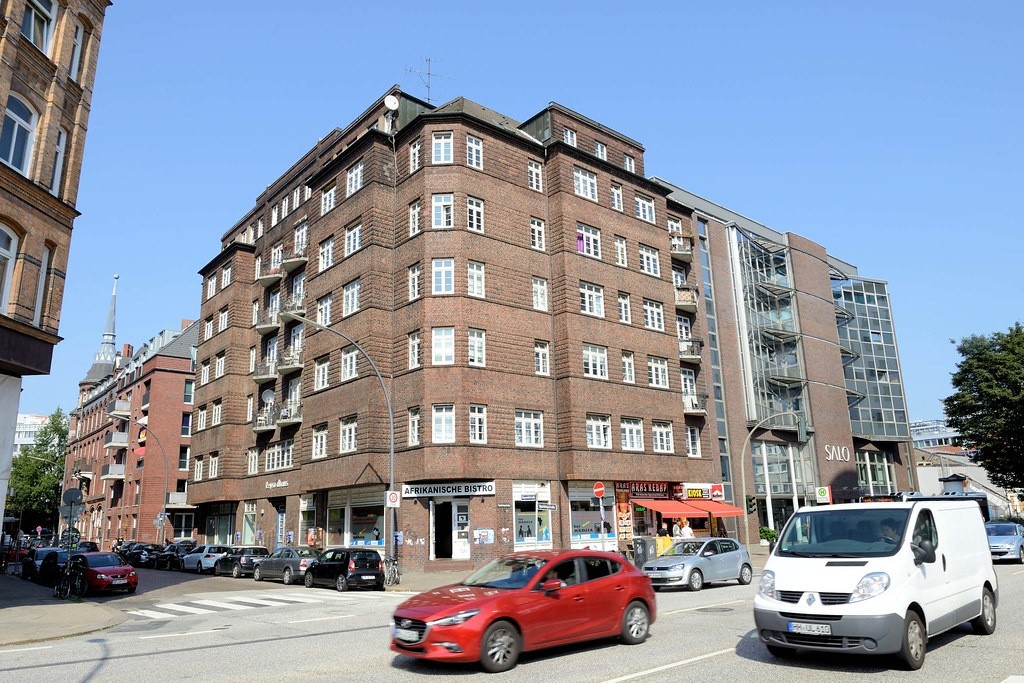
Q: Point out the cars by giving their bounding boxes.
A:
[213,546,271,578]
[69,552,138,596]
[641,537,752,592]
[154,543,197,570]
[21,547,65,583]
[389,549,657,673]
[0,538,164,574]
[253,546,328,585]
[304,547,385,592]
[180,544,232,575]
[39,549,88,588]
[984,521,1024,565]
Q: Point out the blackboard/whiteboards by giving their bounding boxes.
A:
[627,544,634,558]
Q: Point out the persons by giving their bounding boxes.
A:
[657,520,695,539]
[537,568,568,589]
[881,516,901,544]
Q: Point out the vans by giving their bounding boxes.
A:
[753,500,999,671]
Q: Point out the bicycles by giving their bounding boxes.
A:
[381,554,402,587]
[53,558,88,600]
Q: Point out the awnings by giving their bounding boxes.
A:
[632,499,709,519]
[681,500,745,516]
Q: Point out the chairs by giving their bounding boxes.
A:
[824,521,852,542]
[854,521,876,543]
[896,520,921,558]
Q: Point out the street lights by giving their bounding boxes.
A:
[284,311,395,564]
[104,411,169,548]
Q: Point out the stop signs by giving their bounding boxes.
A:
[592,481,607,498]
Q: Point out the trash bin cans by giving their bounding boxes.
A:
[634,538,656,570]
[656,536,672,556]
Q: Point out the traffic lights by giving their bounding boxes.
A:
[797,417,810,443]
